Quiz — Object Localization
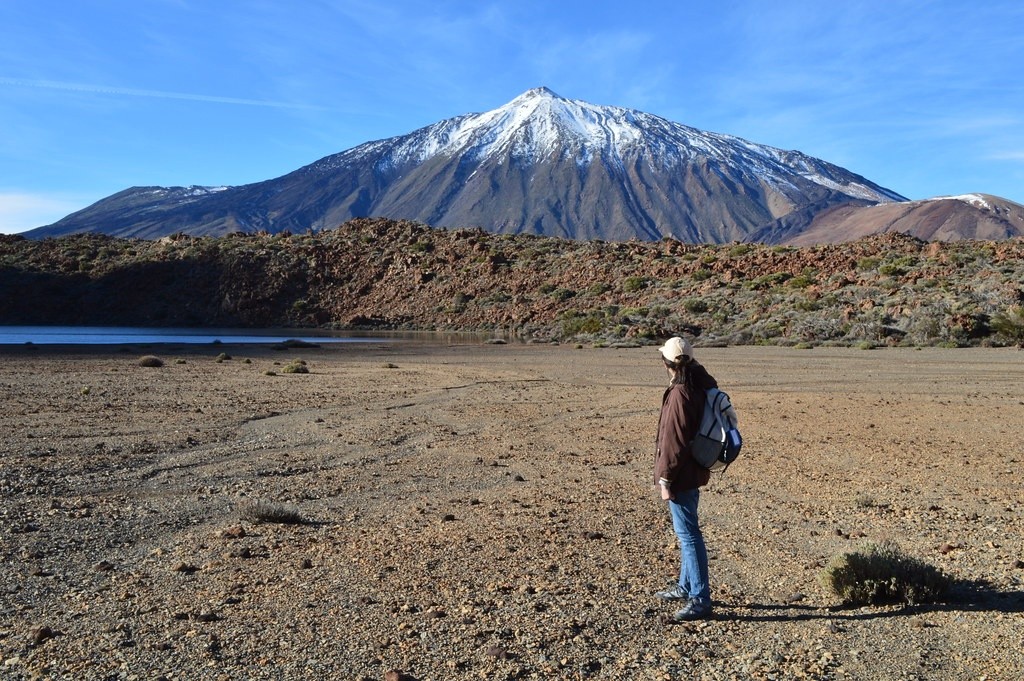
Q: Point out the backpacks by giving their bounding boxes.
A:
[685,385,742,473]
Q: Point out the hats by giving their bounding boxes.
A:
[658,337,693,363]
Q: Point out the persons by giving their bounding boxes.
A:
[652,337,716,619]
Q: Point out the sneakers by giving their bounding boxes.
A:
[675,597,712,620]
[653,588,689,601]
